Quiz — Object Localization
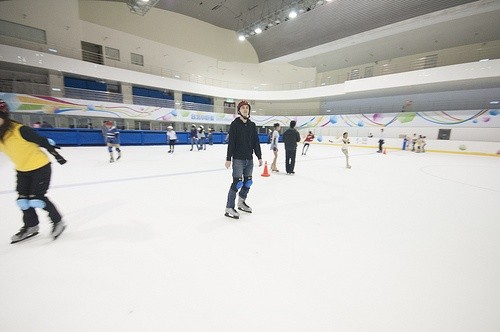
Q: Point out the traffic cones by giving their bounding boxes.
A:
[383,149,386,154]
[260,161,271,177]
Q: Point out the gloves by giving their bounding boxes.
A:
[55,155,66,165]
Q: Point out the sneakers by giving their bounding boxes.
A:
[225,207,239,219]
[10,223,40,245]
[51,219,65,238]
[237,196,252,213]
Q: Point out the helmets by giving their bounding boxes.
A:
[168,126,174,130]
[105,121,113,126]
[237,101,249,115]
[0,99,9,114]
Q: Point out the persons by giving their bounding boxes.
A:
[402,133,427,153]
[166,126,177,153]
[207,128,213,145]
[270,123,281,172]
[302,131,314,156]
[32,122,41,128]
[368,133,373,138]
[342,131,351,168]
[219,129,223,132]
[42,121,53,128]
[102,122,122,163]
[282,121,300,174]
[224,101,263,219]
[188,124,200,151]
[0,99,67,244]
[376,129,384,153]
[197,125,206,150]
